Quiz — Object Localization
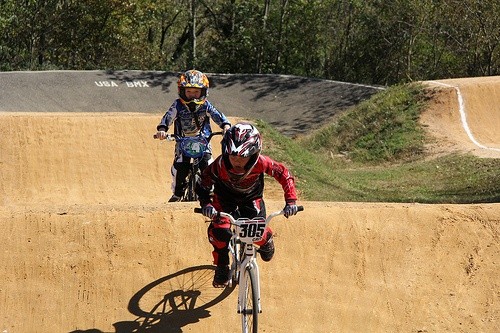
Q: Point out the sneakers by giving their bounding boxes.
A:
[260,234,274,261]
[214,265,229,284]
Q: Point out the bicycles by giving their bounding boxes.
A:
[154,131,223,201]
[195,206,304,333]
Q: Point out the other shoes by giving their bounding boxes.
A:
[169,194,180,202]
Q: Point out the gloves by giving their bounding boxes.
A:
[284,204,298,218]
[202,203,216,219]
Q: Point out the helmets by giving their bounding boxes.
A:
[221,122,262,175]
[177,70,209,114]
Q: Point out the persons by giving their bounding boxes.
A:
[155,70,232,202]
[195,122,298,287]
